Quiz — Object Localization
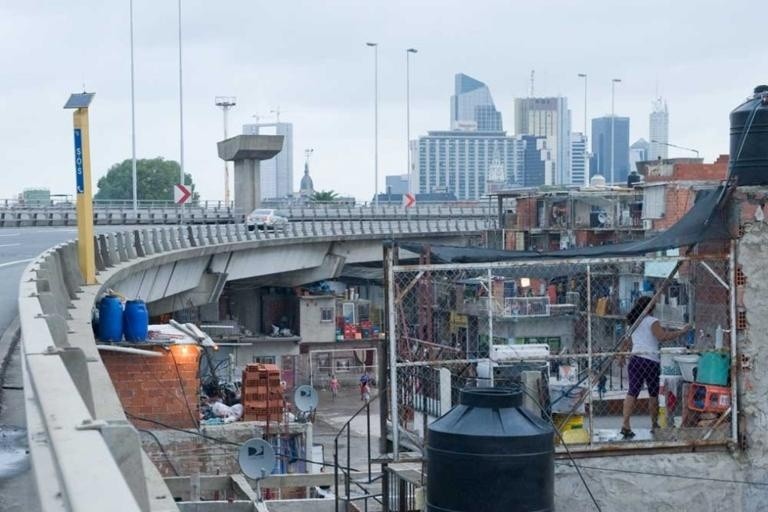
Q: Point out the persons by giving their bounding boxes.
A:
[329,374,339,402]
[619,295,691,436]
[597,372,607,400]
[359,371,369,400]
[527,288,535,315]
[362,381,371,412]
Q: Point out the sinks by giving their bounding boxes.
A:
[674,356,699,383]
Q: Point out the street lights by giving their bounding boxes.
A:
[365,41,379,205]
[406,47,417,192]
[610,79,622,185]
[579,72,589,188]
[214,97,236,209]
[653,141,699,157]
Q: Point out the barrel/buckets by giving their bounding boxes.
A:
[426,387,555,512]
[99,296,123,342]
[660,348,686,375]
[123,300,148,342]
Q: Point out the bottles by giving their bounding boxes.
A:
[688,328,694,344]
[715,324,724,350]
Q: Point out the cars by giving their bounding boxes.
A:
[247,208,289,230]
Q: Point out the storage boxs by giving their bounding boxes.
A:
[547,384,586,414]
[687,383,731,413]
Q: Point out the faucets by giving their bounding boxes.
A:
[699,329,706,340]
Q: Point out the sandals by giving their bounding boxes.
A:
[621,427,635,437]
[650,423,661,433]
